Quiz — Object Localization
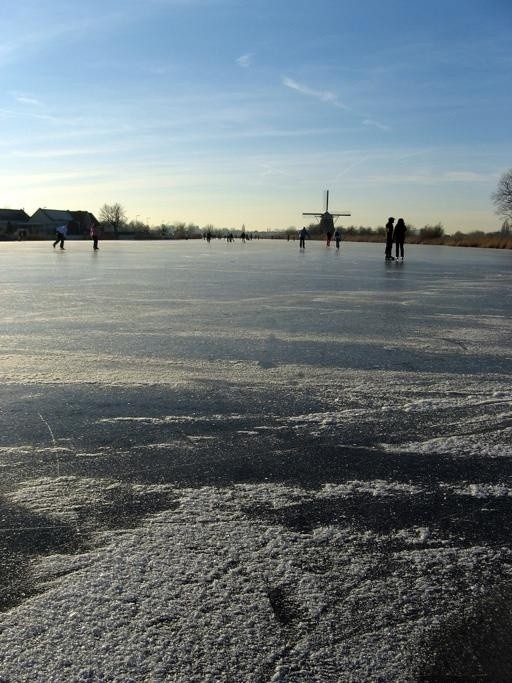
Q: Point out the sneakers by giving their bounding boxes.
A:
[385,256,404,261]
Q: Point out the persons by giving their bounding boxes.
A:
[327,228,331,246]
[298,227,310,248]
[385,218,407,263]
[90,222,99,249]
[203,230,295,243]
[53,222,69,250]
[335,228,341,247]
